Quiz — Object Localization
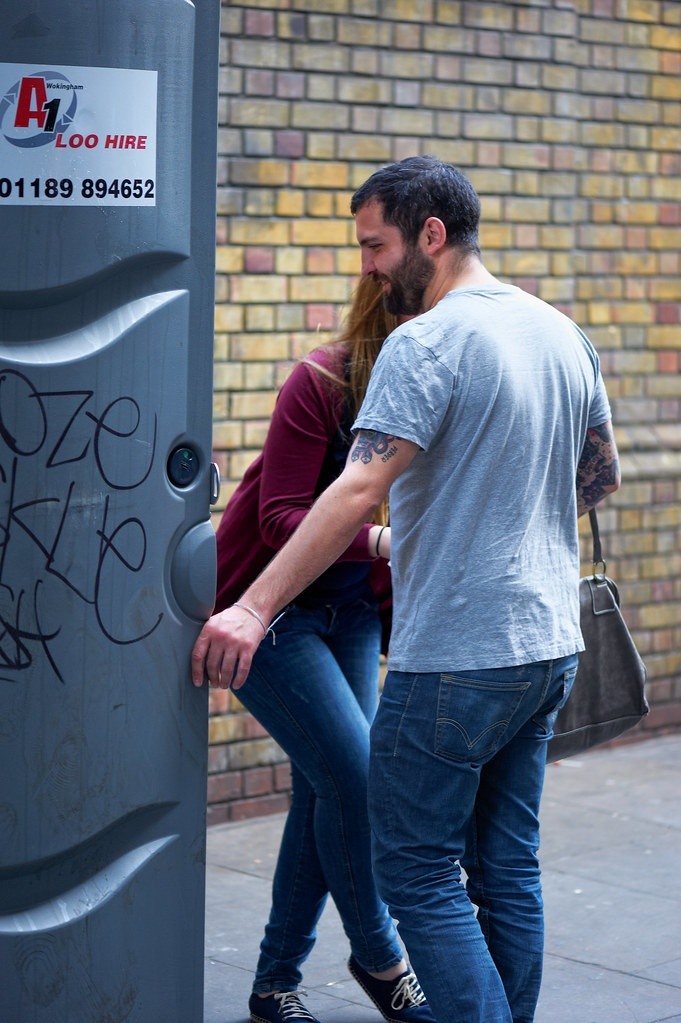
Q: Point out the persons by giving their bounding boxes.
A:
[190,157,624,1023]
[217,266,425,1022]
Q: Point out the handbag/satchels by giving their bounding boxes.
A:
[545,505,649,762]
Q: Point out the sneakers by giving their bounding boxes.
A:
[348,954,438,1023]
[249,989,321,1023]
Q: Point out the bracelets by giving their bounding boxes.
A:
[234,602,286,645]
[376,526,386,556]
